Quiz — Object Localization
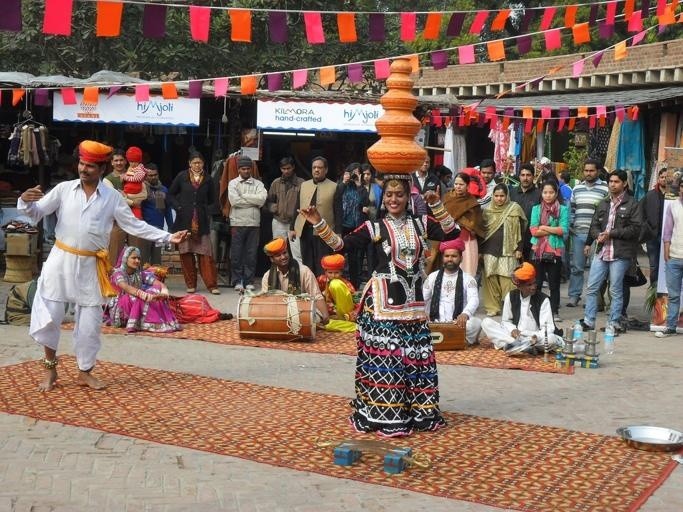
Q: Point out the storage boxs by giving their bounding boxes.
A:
[5,232,38,256]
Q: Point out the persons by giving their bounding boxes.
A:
[360,163,384,280]
[529,180,570,321]
[290,157,338,277]
[104,150,148,269]
[106,246,184,331]
[639,168,668,330]
[654,177,683,338]
[422,241,479,351]
[297,175,461,438]
[510,164,542,260]
[558,171,575,283]
[481,262,564,357]
[409,176,427,225]
[440,173,482,278]
[567,160,610,308]
[17,141,190,392]
[478,183,528,316]
[476,159,501,209]
[317,253,362,331]
[573,170,642,335]
[411,153,440,191]
[268,157,306,267]
[142,164,174,269]
[537,162,558,185]
[431,165,453,186]
[458,168,487,201]
[228,156,268,292]
[238,238,329,333]
[118,146,147,220]
[334,164,370,290]
[167,152,222,294]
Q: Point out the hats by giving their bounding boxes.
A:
[238,155,252,169]
[321,254,345,270]
[264,238,286,257]
[127,147,141,162]
[440,240,465,253]
[511,262,536,287]
[79,140,113,163]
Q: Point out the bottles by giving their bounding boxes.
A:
[605,320,614,355]
[573,319,583,355]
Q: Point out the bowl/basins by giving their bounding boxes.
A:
[616,425,683,453]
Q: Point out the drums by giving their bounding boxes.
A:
[237,295,316,341]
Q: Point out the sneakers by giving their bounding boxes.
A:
[245,284,256,291]
[211,288,221,295]
[187,288,196,294]
[487,311,498,317]
[567,297,579,307]
[234,284,243,291]
[655,327,677,337]
[580,322,594,332]
[553,312,562,322]
[601,323,626,337]
